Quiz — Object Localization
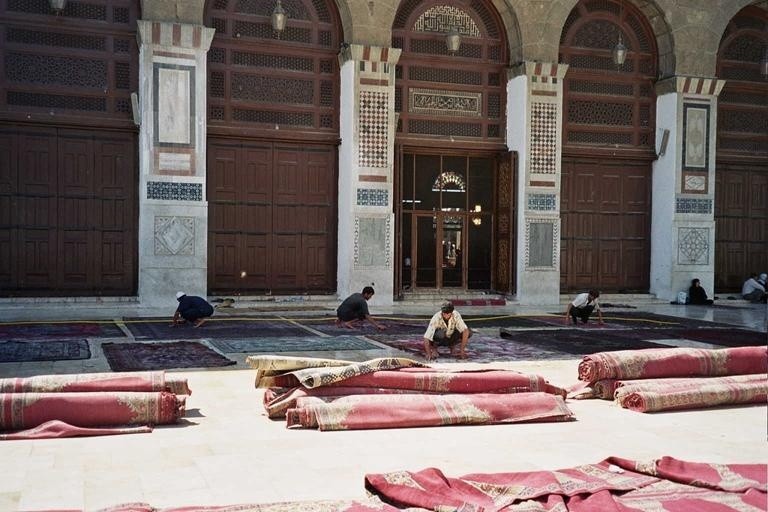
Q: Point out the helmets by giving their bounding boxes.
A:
[175,291,186,300]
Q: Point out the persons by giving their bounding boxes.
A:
[335,285,389,331]
[168,290,215,330]
[423,300,474,360]
[688,278,713,305]
[755,273,768,304]
[565,288,605,326]
[740,270,765,303]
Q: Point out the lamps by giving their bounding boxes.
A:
[444,27,462,55]
[613,33,628,71]
[272,2,286,40]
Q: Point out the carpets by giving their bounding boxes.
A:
[1,299,768,374]
[238,348,577,429]
[356,453,768,510]
[90,497,392,512]
[2,375,198,395]
[1,390,191,444]
[572,344,768,385]
[586,374,768,411]
